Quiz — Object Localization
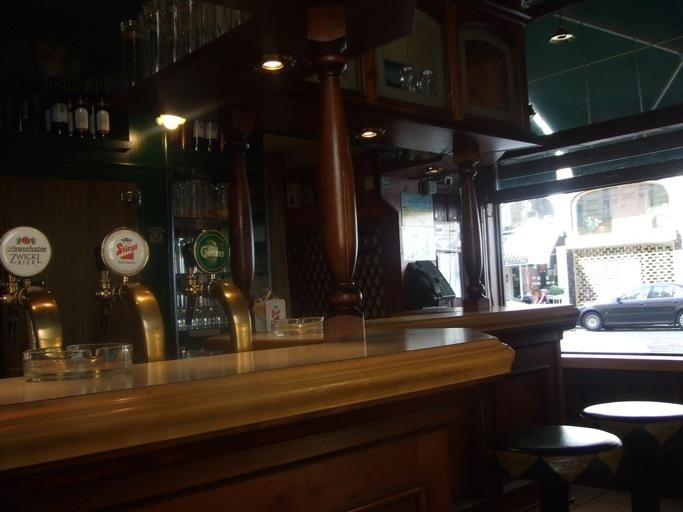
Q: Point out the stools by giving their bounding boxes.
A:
[581,400,683,510]
[491,423,623,511]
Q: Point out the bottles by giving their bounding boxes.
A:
[166,116,225,168]
[1,71,115,141]
[178,169,248,332]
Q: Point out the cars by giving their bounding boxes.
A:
[573,282,683,330]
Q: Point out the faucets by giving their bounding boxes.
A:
[183,241,253,352]
[0,269,64,361]
[92,242,165,361]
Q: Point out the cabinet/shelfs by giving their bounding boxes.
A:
[336,0,530,140]
[174,213,230,297]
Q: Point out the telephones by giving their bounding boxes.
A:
[407,260,455,308]
